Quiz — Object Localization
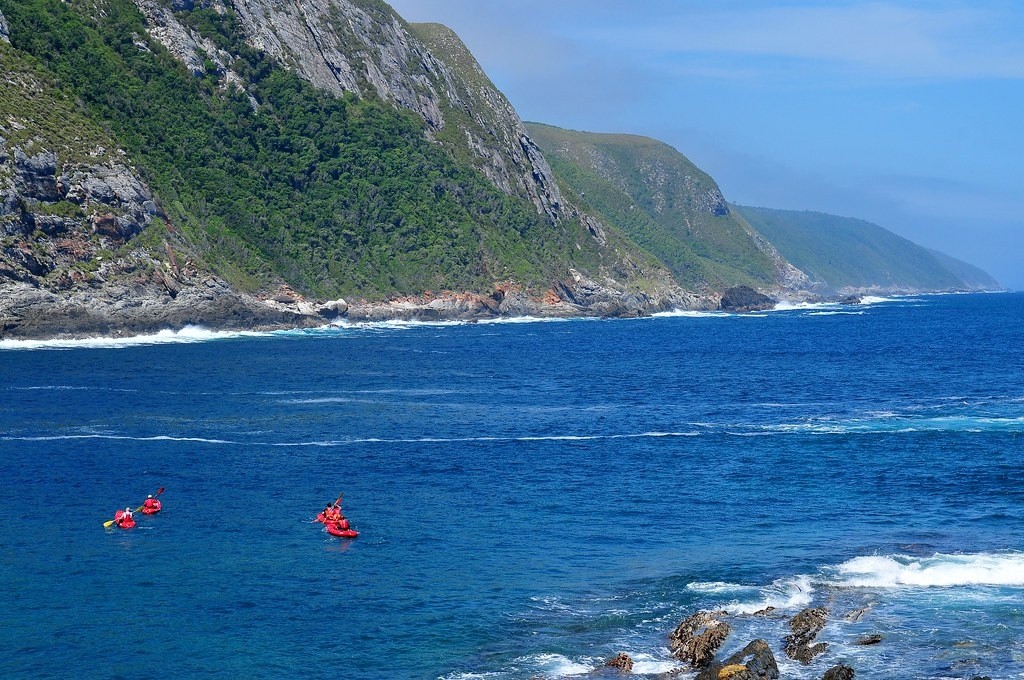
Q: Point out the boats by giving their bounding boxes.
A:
[317,513,358,538]
[142,499,162,515]
[114,510,136,529]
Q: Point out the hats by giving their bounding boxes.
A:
[126,507,130,511]
[148,495,153,498]
[327,502,331,506]
[337,514,342,519]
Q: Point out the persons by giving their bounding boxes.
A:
[117,507,134,522]
[322,502,349,530]
[144,494,157,508]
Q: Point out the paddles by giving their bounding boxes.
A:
[153,487,164,499]
[103,506,144,527]
[312,491,345,526]
